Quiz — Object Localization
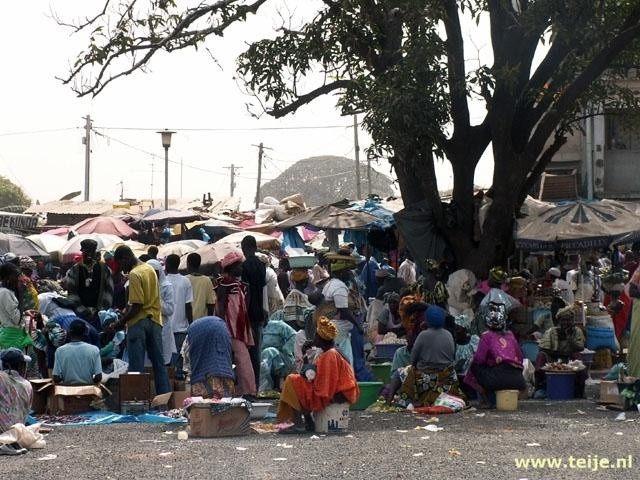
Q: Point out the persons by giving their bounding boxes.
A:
[0,235,640,458]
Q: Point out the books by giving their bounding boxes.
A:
[186,402,251,437]
[599,380,620,402]
[545,371,584,401]
[350,344,407,409]
[119,373,151,403]
[150,390,188,410]
[29,378,112,414]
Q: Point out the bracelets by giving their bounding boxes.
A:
[373,343,405,361]
[347,382,382,410]
[288,256,318,269]
[367,362,395,382]
[246,401,272,420]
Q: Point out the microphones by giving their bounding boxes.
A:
[496,390,519,411]
[314,399,350,435]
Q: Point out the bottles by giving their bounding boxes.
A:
[549,266,561,277]
[0,347,31,362]
[81,239,98,252]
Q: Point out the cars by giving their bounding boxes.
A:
[544,372,576,401]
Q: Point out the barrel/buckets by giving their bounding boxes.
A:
[0,442,27,455]
[278,426,306,434]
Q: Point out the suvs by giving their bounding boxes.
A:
[494,390,519,411]
[572,350,596,380]
[312,402,350,435]
[539,364,587,401]
